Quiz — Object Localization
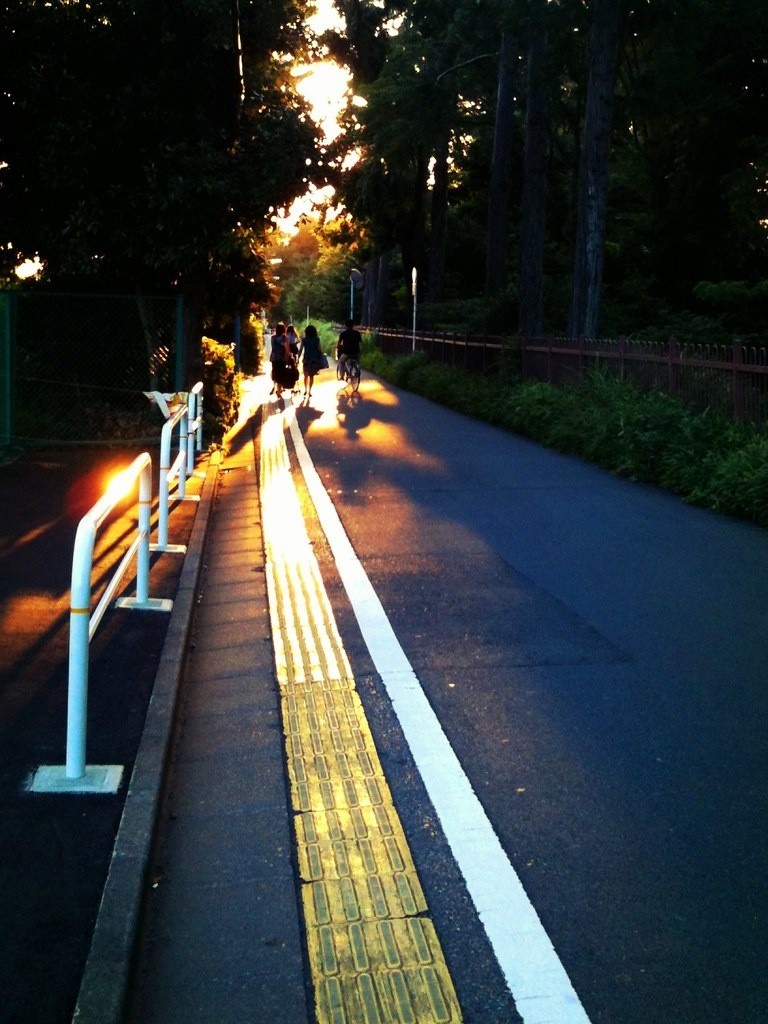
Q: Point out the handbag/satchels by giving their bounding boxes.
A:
[275,357,299,388]
[319,352,329,369]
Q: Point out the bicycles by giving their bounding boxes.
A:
[336,344,361,393]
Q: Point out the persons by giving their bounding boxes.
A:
[269,321,300,400]
[298,325,323,397]
[336,318,363,382]
